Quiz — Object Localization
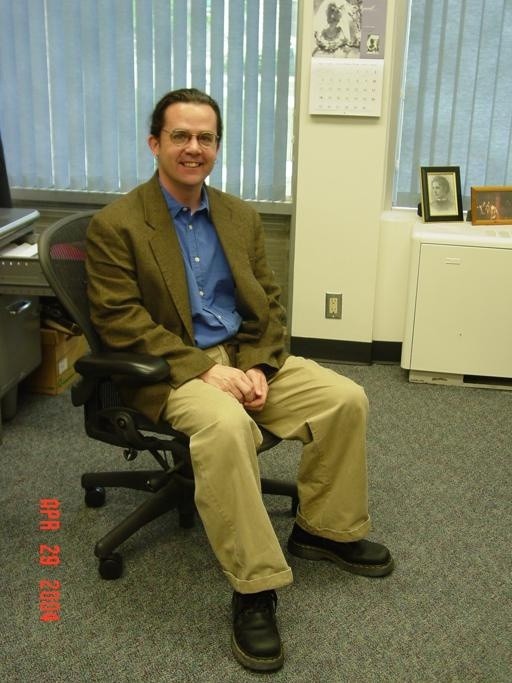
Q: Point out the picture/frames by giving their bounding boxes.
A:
[471,186,512,225]
[420,166,463,222]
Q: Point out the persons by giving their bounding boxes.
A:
[82,86,396,674]
[429,175,455,213]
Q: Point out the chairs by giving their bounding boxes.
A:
[38,209,298,580]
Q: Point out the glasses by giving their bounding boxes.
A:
[162,126,221,150]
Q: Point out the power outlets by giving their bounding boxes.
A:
[326,293,342,320]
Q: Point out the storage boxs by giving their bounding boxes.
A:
[33,328,89,395]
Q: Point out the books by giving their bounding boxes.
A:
[0,241,42,261]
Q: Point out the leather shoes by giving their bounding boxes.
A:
[287,524,395,577]
[231,588,284,672]
